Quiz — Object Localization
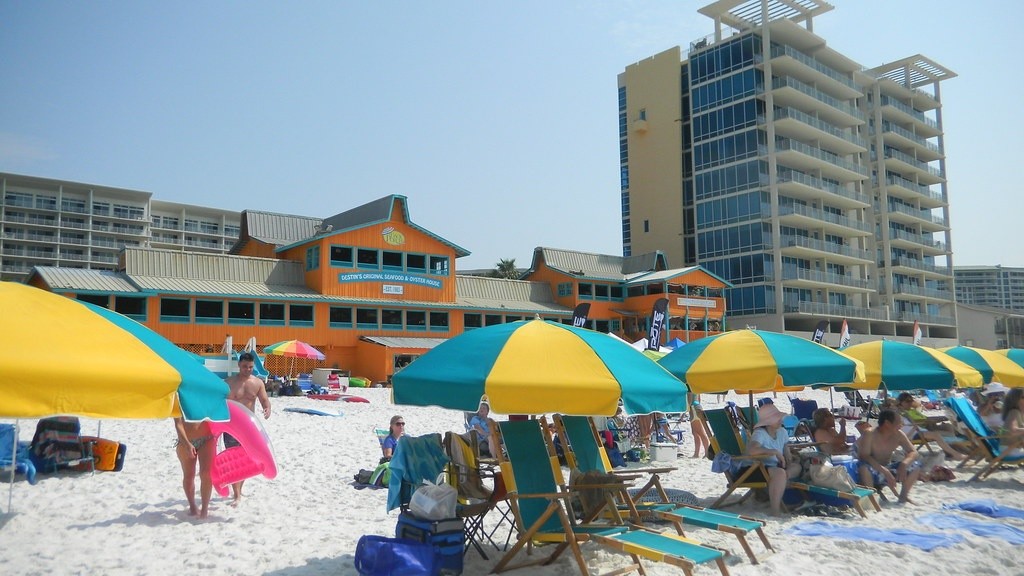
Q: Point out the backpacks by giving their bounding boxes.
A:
[369,462,392,488]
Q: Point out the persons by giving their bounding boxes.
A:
[173,418,217,519]
[383,415,405,459]
[468,403,502,459]
[222,353,271,506]
[596,385,1024,516]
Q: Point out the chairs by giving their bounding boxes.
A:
[372,386,1024,576]
[37,415,96,477]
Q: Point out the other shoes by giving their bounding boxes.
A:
[677,452,684,457]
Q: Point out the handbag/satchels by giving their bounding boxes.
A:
[353,469,382,485]
[603,440,628,469]
[409,472,457,521]
[626,448,641,461]
[809,463,856,493]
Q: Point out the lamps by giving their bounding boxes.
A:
[325,225,333,232]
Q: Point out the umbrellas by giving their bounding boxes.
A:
[657,327,865,436]
[0,280,230,515]
[934,345,1024,389]
[811,337,982,405]
[263,339,325,386]
[391,313,689,422]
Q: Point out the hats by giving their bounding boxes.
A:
[980,381,1012,398]
[754,403,788,428]
[459,473,494,498]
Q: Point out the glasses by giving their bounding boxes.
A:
[989,392,1005,396]
[395,422,405,426]
[904,399,916,403]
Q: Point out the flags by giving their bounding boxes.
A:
[912,319,922,345]
[839,318,850,349]
[812,321,830,343]
[647,298,669,350]
[573,303,590,327]
